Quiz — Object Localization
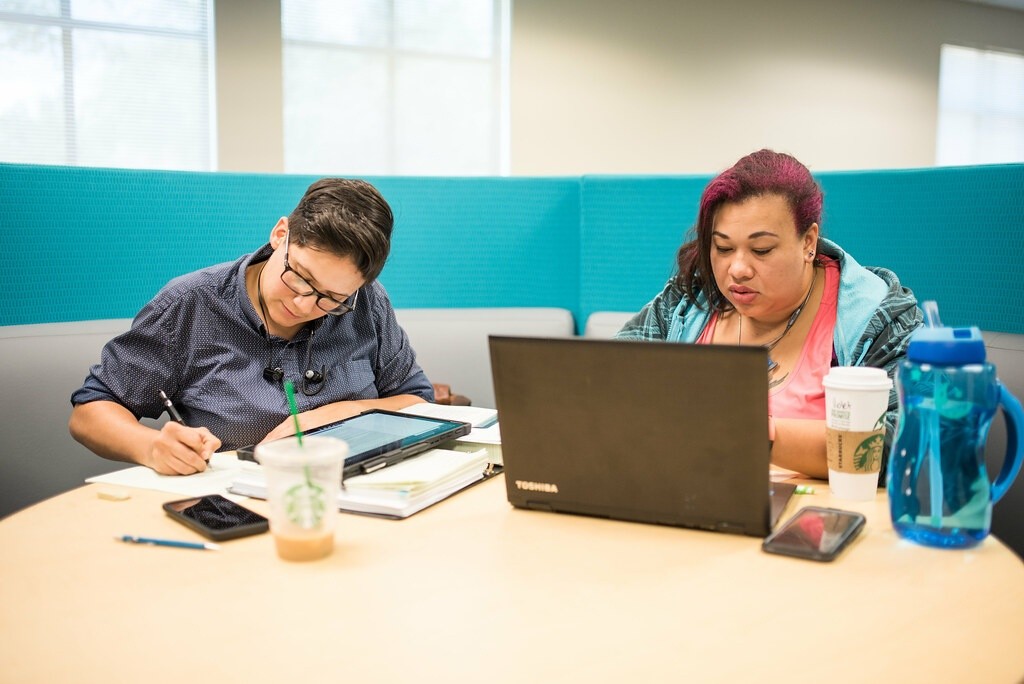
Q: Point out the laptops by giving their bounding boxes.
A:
[488,335,798,539]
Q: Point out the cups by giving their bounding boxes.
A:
[820,365,891,514]
[253,436,347,562]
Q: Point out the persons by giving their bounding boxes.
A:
[68,177,434,476]
[613,149,927,489]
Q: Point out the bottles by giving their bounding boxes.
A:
[888,325,1024,545]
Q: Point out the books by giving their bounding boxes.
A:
[226,449,489,519]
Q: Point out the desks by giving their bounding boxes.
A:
[0,464,1024,684]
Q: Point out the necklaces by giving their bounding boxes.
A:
[739,268,817,370]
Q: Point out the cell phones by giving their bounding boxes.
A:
[762,506,866,562]
[162,494,269,543]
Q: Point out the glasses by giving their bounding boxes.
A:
[280,230,360,316]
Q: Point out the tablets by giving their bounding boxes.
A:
[237,408,471,482]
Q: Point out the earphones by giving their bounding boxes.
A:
[305,370,323,384]
[264,368,284,382]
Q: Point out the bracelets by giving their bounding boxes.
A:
[768,414,775,450]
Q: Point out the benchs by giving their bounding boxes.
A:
[0,306,575,523]
[588,310,1024,565]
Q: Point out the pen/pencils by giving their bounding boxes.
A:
[157,388,215,471]
[792,485,815,495]
[114,535,224,553]
